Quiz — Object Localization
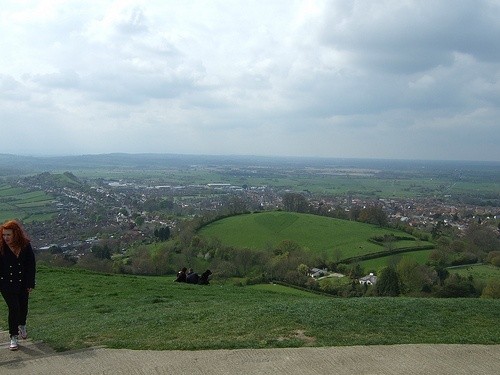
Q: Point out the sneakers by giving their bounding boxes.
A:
[10,335,18,350]
[19,324,28,339]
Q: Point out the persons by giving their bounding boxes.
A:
[186,268,199,284]
[0,220,36,352]
[173,267,187,283]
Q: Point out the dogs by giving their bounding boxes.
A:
[199,270,212,285]
